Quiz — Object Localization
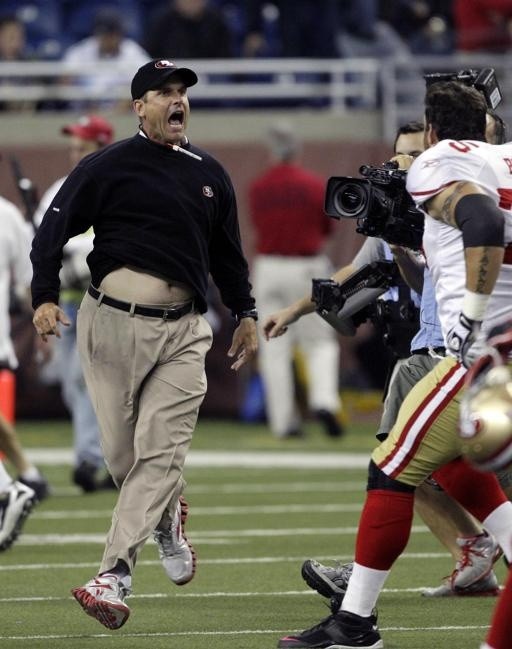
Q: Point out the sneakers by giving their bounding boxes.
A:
[301,559,354,610]
[0,476,48,552]
[277,601,384,649]
[152,496,196,585]
[71,576,131,630]
[421,561,501,597]
[454,529,503,590]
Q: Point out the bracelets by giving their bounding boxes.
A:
[462,286,491,324]
[235,310,260,323]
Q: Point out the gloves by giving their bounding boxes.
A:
[446,312,483,369]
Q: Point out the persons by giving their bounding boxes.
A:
[480,567,511,649]
[247,124,347,444]
[29,59,261,632]
[1,0,511,111]
[276,80,512,649]
[0,193,51,553]
[32,114,117,491]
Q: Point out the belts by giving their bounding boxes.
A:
[88,284,197,321]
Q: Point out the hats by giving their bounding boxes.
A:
[131,58,198,100]
[61,116,114,147]
